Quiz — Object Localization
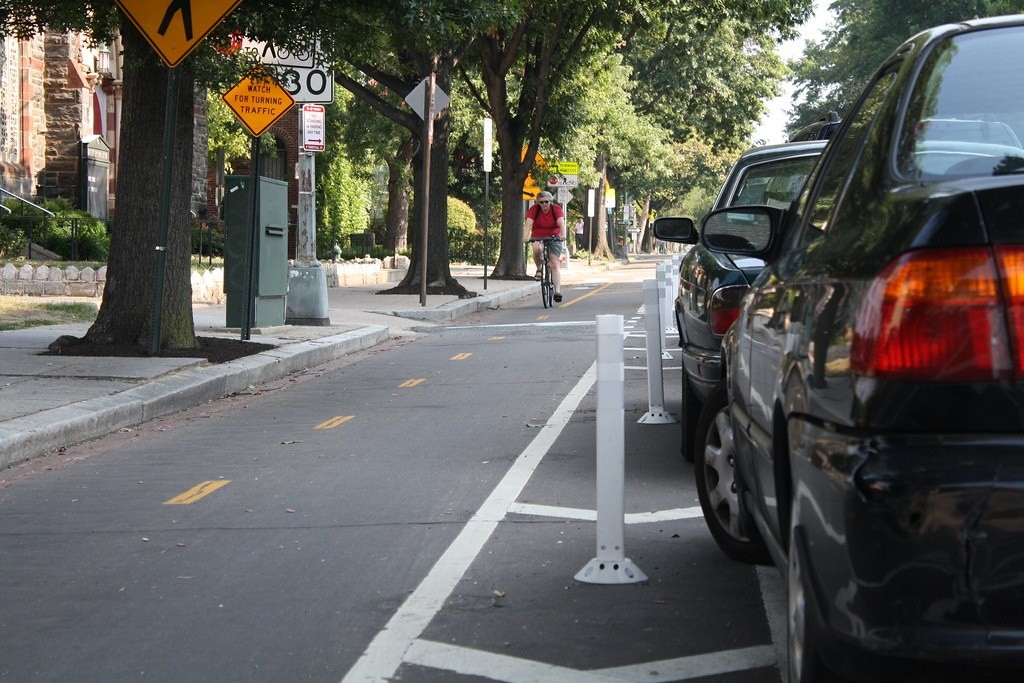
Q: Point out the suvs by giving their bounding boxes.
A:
[762,107,1024,210]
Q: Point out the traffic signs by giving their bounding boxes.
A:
[303,105,325,152]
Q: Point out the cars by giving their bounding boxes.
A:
[652,139,1024,461]
[694,16,1024,683]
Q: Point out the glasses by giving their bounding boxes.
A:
[538,200,550,204]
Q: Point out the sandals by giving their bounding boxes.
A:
[534,270,543,281]
[553,291,563,302]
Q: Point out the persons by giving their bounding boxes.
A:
[574,217,585,249]
[524,192,567,303]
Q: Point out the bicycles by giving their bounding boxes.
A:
[527,236,562,309]
